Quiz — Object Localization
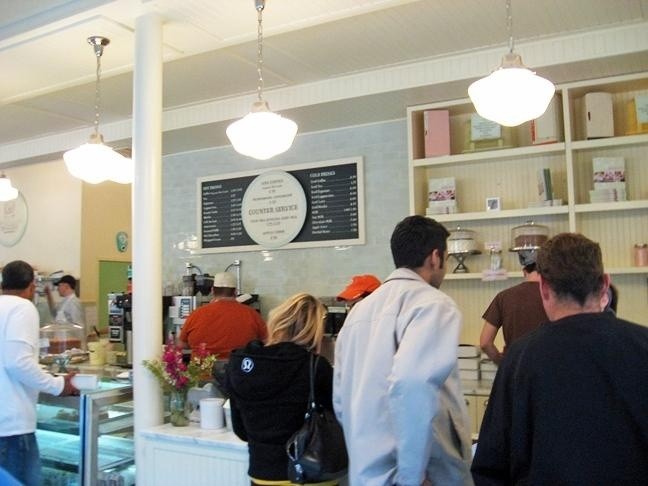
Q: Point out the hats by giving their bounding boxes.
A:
[336,276,381,302]
[53,275,76,289]
[214,273,235,288]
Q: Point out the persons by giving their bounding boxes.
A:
[335,274,381,313]
[44,275,87,352]
[0,260,80,486]
[179,272,268,399]
[469,232,648,486]
[226,293,348,486]
[479,262,550,367]
[332,215,475,486]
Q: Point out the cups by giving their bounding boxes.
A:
[200,397,225,428]
[86,342,106,365]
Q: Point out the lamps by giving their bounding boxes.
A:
[466,0,555,129]
[222,0,301,165]
[59,34,126,184]
[0,159,20,205]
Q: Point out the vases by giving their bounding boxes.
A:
[167,387,191,427]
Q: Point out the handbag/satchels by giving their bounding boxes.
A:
[286,411,348,482]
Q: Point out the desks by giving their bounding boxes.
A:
[136,420,252,486]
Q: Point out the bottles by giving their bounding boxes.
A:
[633,243,648,267]
[488,249,502,271]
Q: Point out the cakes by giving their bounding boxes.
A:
[447,236,478,255]
[49,338,81,354]
[510,233,548,249]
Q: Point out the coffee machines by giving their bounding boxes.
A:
[168,295,261,357]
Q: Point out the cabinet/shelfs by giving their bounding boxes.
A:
[403,67,647,445]
[35,367,136,486]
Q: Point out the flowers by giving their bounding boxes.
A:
[144,338,217,421]
